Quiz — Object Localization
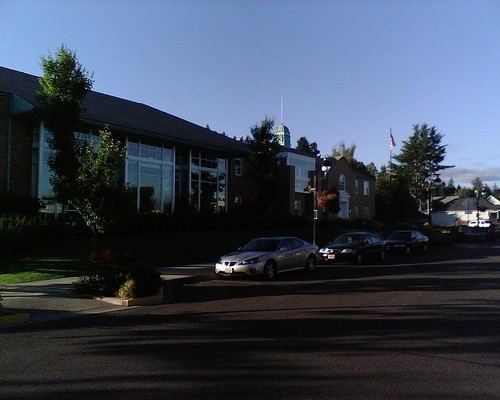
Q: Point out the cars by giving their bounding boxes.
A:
[383,230,430,256]
[319,231,389,264]
[214,236,320,281]
